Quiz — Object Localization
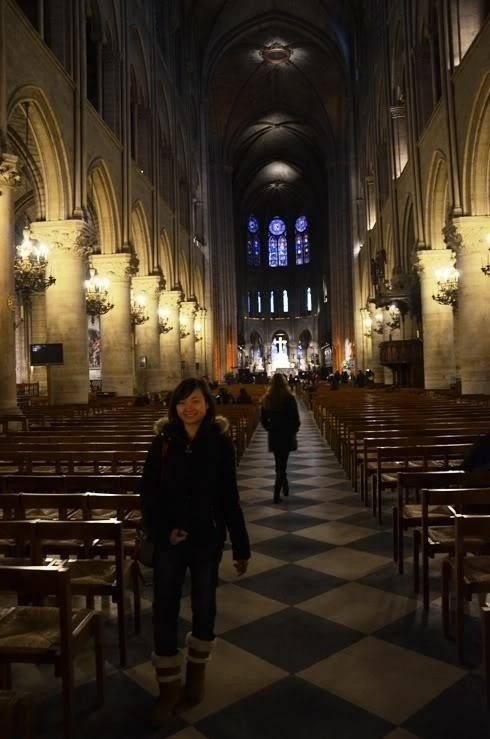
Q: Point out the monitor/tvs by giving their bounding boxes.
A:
[29,343,64,365]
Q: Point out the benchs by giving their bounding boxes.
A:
[296,381,490,690]
[1,384,268,738]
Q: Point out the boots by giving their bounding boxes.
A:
[144,646,182,724]
[271,479,293,504]
[181,650,209,712]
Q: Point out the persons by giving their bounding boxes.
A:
[138,378,252,728]
[257,373,301,504]
[459,432,490,515]
[288,369,365,392]
[134,373,267,406]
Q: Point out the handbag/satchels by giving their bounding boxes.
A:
[136,529,156,568]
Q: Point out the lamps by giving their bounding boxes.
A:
[386,306,401,330]
[482,258,489,276]
[180,323,190,339]
[194,329,203,342]
[364,321,373,337]
[12,236,55,295]
[373,315,386,334]
[82,274,116,317]
[432,268,459,307]
[158,312,174,334]
[129,296,149,326]
